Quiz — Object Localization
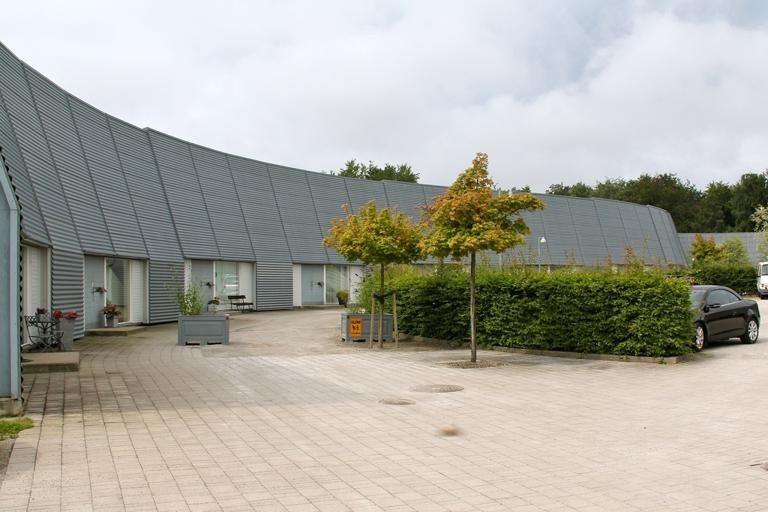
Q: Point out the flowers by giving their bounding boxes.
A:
[100,299,121,319]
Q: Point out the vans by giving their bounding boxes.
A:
[756,260,768,297]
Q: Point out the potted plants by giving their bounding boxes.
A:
[207,299,219,313]
[176,282,230,346]
[340,288,394,343]
[336,290,350,308]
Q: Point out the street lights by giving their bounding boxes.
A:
[537,235,547,269]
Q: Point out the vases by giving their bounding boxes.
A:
[106,314,118,328]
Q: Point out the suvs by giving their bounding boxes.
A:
[220,274,240,299]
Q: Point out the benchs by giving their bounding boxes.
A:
[23,316,65,352]
[228,295,253,312]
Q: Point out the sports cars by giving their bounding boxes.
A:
[689,283,761,352]
